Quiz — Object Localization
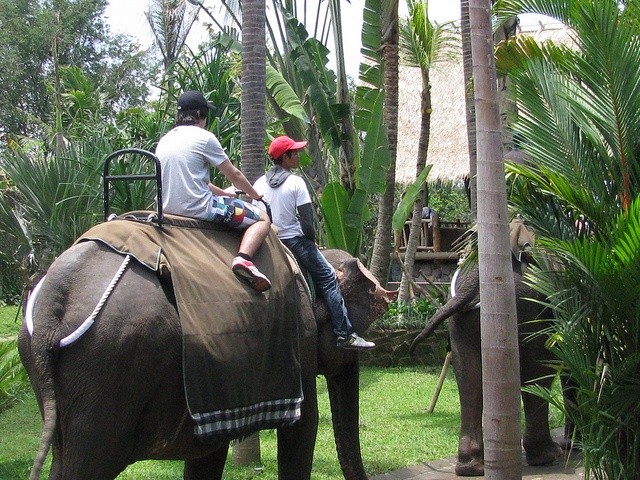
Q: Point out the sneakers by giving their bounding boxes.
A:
[231,255,271,293]
[336,331,376,350]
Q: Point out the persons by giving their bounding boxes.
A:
[252,135,377,351]
[154,90,273,294]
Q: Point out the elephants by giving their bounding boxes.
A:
[17,219,399,480]
[409,226,603,475]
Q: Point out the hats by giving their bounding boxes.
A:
[177,91,217,111]
[269,136,307,161]
